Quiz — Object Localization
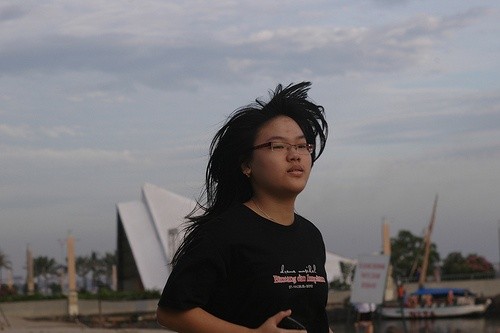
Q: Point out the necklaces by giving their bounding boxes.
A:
[250,198,294,222]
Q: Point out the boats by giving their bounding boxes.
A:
[378,287,492,317]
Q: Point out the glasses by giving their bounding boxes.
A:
[251,140,314,155]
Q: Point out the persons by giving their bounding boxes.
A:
[16,238,499,310]
[157,81,333,333]
